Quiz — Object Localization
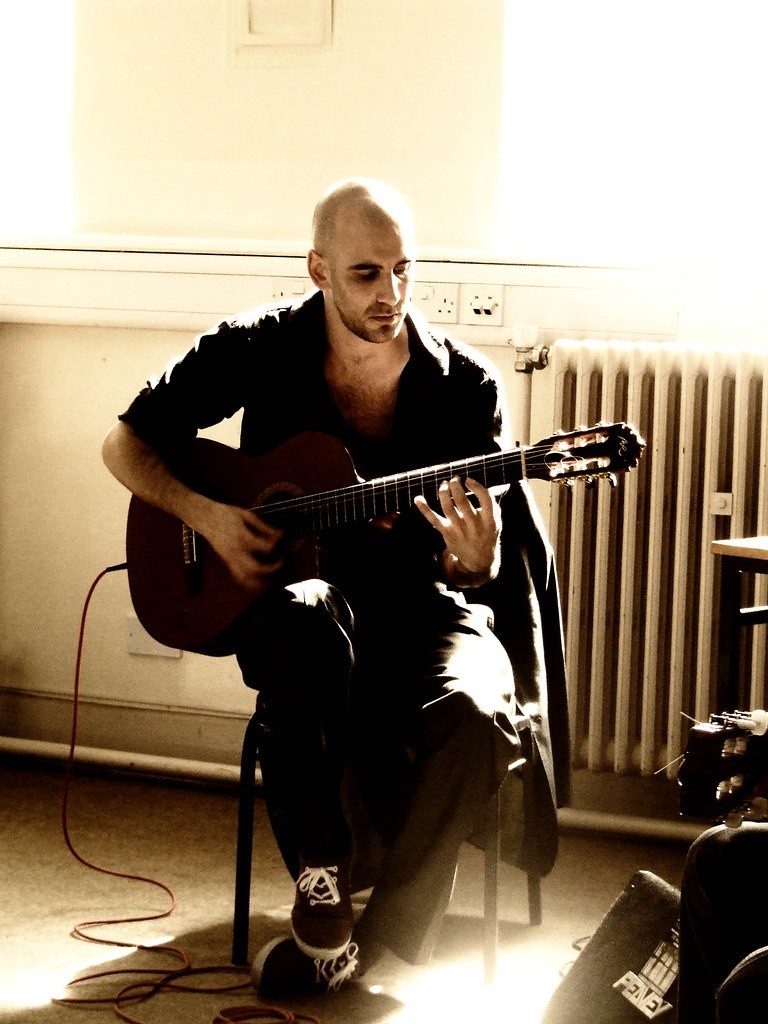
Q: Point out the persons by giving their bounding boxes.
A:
[100,176,515,1000]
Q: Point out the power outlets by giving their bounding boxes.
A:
[462,285,503,326]
[410,285,457,324]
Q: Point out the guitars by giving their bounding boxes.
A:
[124,420,648,658]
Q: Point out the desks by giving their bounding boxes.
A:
[711,535,768,715]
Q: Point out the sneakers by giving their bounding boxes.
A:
[291,845,355,960]
[251,934,362,1004]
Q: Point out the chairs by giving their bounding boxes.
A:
[232,477,574,972]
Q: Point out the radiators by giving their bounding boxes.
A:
[543,342,768,844]
